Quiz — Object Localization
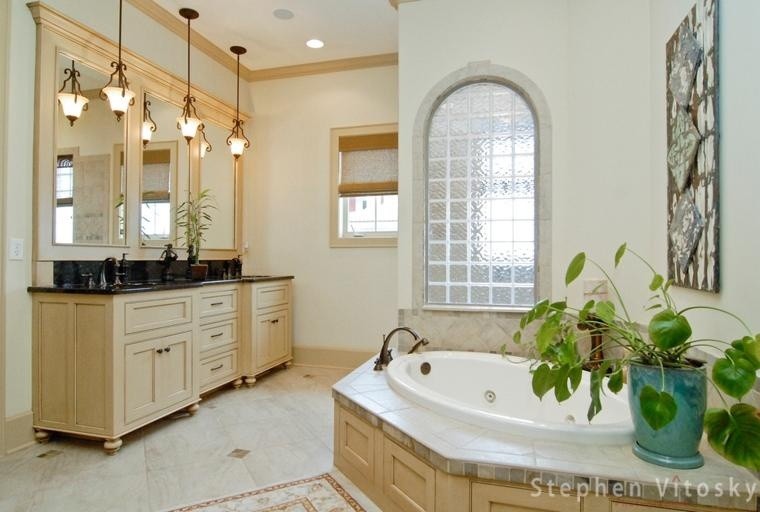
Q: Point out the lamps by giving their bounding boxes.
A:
[225,46,250,159]
[98,0,136,123]
[574,279,612,374]
[57,60,90,127]
[142,97,156,148]
[200,132,211,161]
[175,8,202,145]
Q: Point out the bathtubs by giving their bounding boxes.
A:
[382,348,634,447]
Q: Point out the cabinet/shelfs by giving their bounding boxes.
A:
[240,278,296,389]
[30,287,200,454]
[199,284,240,399]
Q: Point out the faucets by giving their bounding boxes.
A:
[228,258,240,278]
[374,327,418,371]
[407,337,430,354]
[100,257,120,286]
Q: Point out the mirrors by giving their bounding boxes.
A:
[138,86,193,252]
[198,116,238,251]
[52,44,128,248]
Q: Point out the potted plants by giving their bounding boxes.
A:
[500,237,760,477]
[173,188,215,280]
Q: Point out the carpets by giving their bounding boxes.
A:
[159,461,367,512]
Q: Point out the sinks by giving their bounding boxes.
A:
[110,283,162,291]
[231,276,271,278]
